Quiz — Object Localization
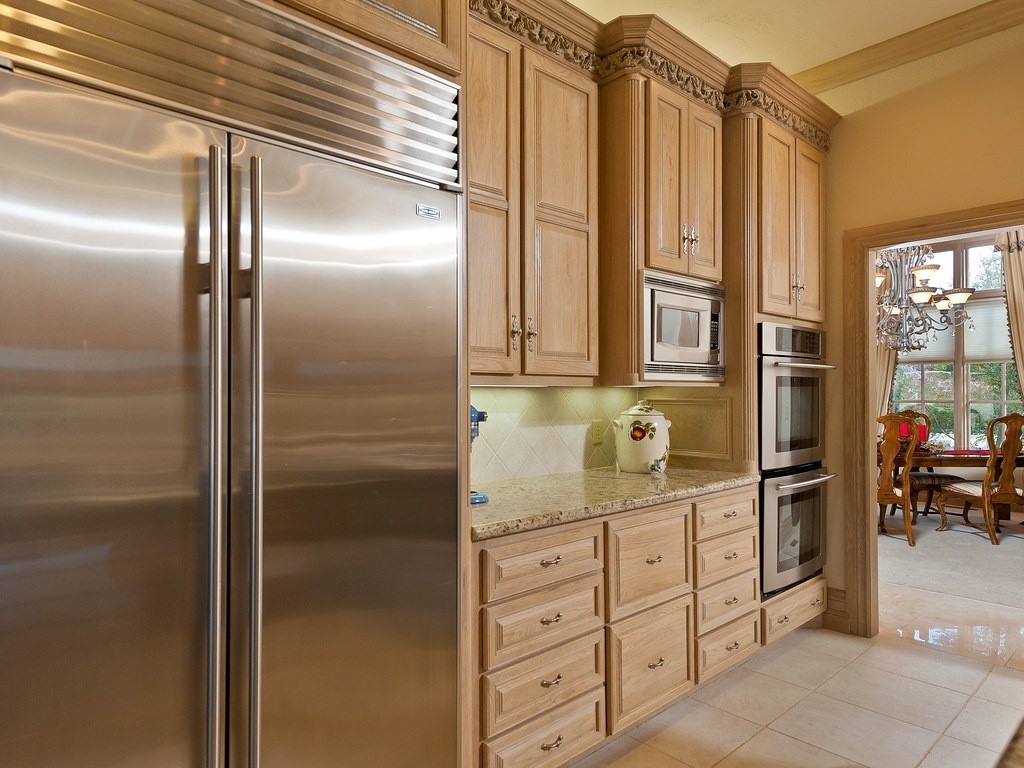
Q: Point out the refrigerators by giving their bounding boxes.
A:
[0,0,461,768]
[2,58,469,768]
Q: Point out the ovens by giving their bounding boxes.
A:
[759,321,839,594]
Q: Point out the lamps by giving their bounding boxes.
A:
[875,244,976,356]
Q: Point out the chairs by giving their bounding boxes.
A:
[936,412,1024,545]
[876,414,918,547]
[888,409,973,525]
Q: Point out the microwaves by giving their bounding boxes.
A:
[638,268,726,382]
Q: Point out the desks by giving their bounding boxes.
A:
[875,452,1024,525]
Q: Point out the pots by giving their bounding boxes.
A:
[613,401,672,473]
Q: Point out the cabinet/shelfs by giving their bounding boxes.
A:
[464,0,841,768]
